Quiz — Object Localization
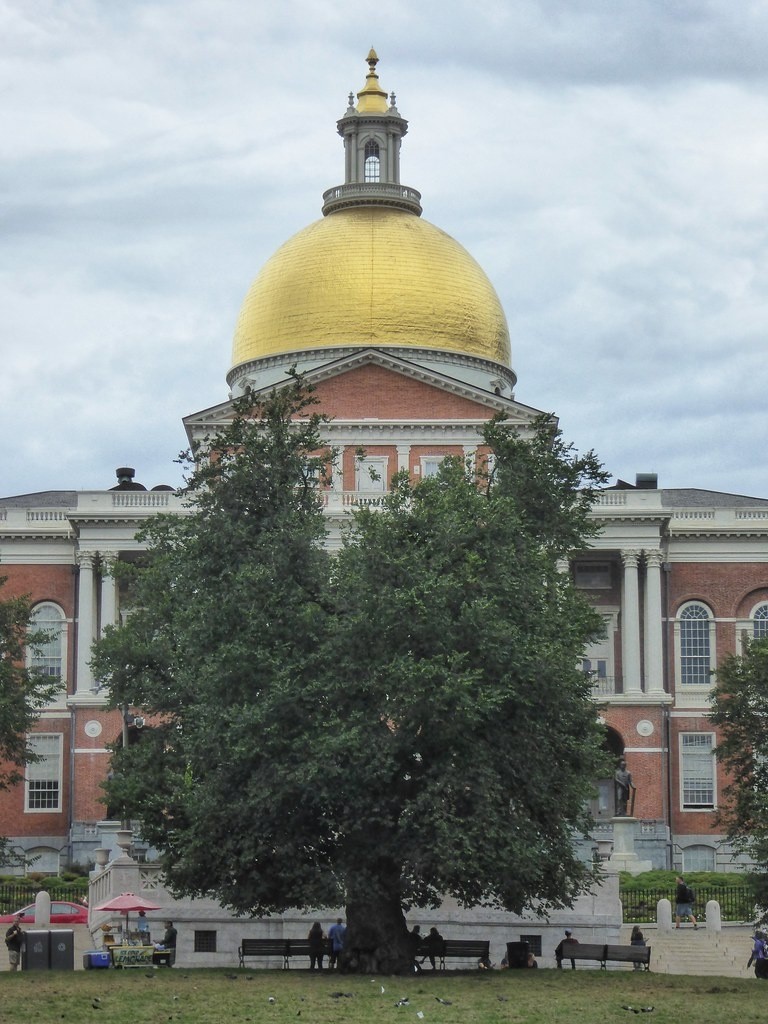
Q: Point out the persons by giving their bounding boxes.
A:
[614,761,635,816]
[408,925,422,961]
[153,921,177,968]
[524,953,538,968]
[630,925,650,971]
[419,927,444,970]
[673,876,699,931]
[555,928,580,970]
[4,918,23,971]
[750,930,768,980]
[326,918,346,969]
[479,952,495,969]
[308,921,327,970]
[500,952,509,969]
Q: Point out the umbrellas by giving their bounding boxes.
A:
[747,953,754,970]
[92,893,163,930]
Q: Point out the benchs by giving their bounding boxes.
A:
[238,939,339,971]
[560,943,652,972]
[416,937,492,970]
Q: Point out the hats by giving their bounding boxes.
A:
[139,910,146,914]
[565,929,573,934]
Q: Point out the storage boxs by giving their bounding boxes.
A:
[83,951,112,969]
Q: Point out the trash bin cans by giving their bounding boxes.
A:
[49,929,75,970]
[21,930,50,970]
[507,941,529,968]
